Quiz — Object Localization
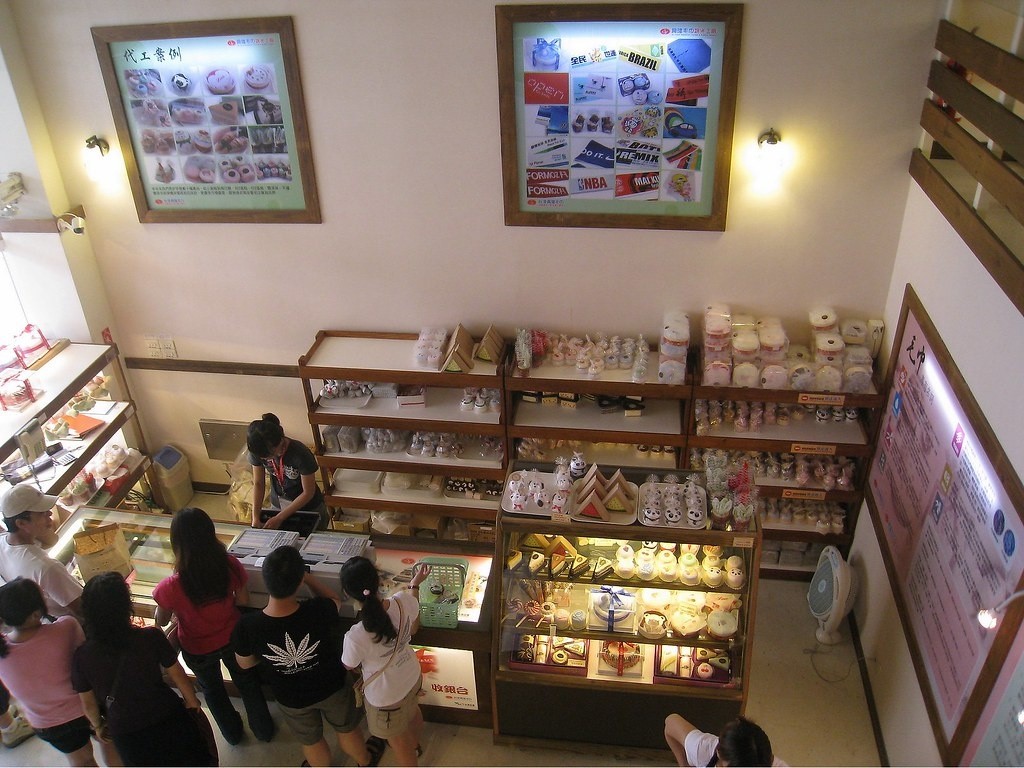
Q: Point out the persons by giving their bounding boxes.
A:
[246,412,330,530]
[664,714,789,767]
[229,545,386,767]
[339,555,432,767]
[0,483,123,767]
[152,507,275,745]
[71,570,219,767]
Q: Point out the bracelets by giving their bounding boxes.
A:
[90,715,106,730]
[408,584,420,590]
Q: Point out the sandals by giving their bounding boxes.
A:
[358,735,386,766]
[301,760,310,767]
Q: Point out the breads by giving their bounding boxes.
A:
[520,390,645,416]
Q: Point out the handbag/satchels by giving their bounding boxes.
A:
[73,522,132,585]
[353,677,366,708]
[155,614,181,658]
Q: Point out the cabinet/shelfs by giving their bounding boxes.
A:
[0,342,168,554]
[298,330,886,581]
[490,458,763,762]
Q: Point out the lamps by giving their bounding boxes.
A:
[83,136,109,164]
[759,127,782,158]
[977,591,1024,629]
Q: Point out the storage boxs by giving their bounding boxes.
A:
[466,519,496,543]
[409,513,446,540]
[327,507,371,534]
[369,516,409,536]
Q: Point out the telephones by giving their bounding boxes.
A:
[14,418,46,465]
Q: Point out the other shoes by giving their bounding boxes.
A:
[387,740,423,757]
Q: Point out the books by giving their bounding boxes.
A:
[44,413,105,441]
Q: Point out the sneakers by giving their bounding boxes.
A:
[0,705,35,748]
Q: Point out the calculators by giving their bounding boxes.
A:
[52,448,77,465]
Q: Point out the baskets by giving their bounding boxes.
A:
[411,556,468,629]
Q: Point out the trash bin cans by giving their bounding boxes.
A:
[150,444,194,513]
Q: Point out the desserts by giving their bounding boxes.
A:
[320,380,503,496]
[57,445,126,506]
[506,534,746,680]
[509,437,704,528]
[693,399,860,535]
[551,332,650,384]
[703,304,880,392]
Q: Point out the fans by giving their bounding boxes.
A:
[807,546,860,645]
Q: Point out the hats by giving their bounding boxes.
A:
[0,483,58,520]
